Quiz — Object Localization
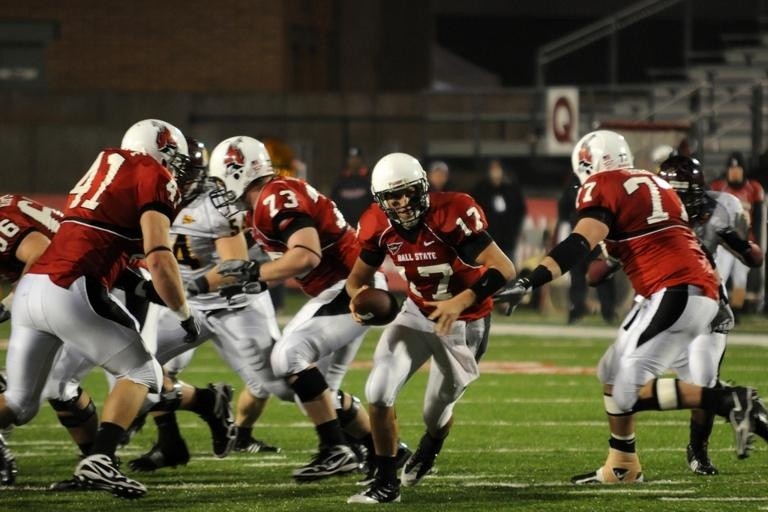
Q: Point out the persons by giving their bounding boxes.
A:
[584,153,768,476]
[471,157,528,314]
[345,152,517,504]
[1,120,201,498]
[558,171,617,323]
[207,133,410,478]
[113,138,279,471]
[330,145,377,230]
[707,151,767,323]
[430,157,453,193]
[489,129,757,487]
[0,191,235,487]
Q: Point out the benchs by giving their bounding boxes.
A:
[597,36,768,184]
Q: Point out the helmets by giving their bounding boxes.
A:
[571,130,705,224]
[119,117,276,205]
[370,152,432,232]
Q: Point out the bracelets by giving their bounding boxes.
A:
[469,267,506,305]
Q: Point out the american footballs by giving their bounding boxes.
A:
[354,288,400,326]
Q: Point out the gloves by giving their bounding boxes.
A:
[142,258,268,345]
[704,299,735,334]
[491,272,535,317]
[714,224,748,254]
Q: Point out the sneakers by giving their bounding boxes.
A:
[291,431,445,505]
[50,479,90,492]
[72,451,147,499]
[128,437,190,473]
[0,434,18,487]
[569,384,768,487]
[198,382,234,459]
[231,426,282,456]
